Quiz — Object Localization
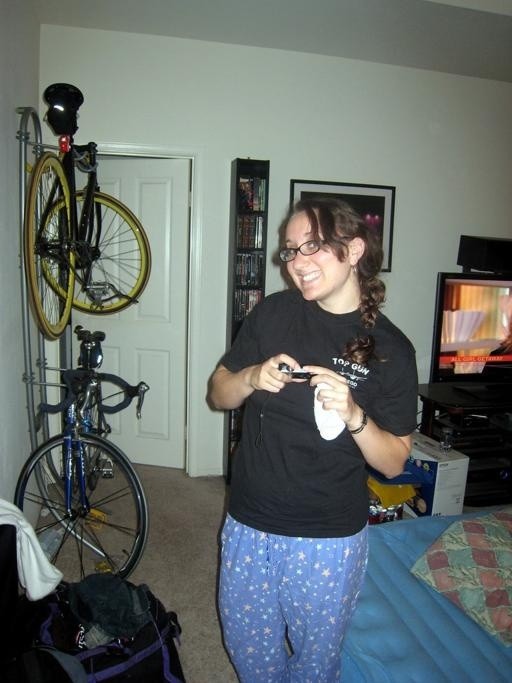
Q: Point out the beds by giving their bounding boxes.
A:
[332,509,512,682]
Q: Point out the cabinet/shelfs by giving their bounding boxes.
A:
[414,381,512,502]
[222,157,271,485]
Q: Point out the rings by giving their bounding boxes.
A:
[268,355,279,368]
[331,389,337,399]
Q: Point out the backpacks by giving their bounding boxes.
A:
[36,573,186,682]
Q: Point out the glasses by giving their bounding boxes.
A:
[280,241,327,261]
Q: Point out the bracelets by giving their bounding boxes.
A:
[348,409,369,435]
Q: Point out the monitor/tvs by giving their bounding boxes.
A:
[429,271,512,383]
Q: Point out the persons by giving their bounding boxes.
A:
[202,192,420,683]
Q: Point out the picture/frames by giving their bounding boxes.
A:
[289,178,395,274]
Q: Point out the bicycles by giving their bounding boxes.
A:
[14,325,150,582]
[19,83,155,341]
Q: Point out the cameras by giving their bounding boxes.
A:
[278,364,314,381]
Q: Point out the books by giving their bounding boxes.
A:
[229,397,246,454]
[232,173,269,323]
